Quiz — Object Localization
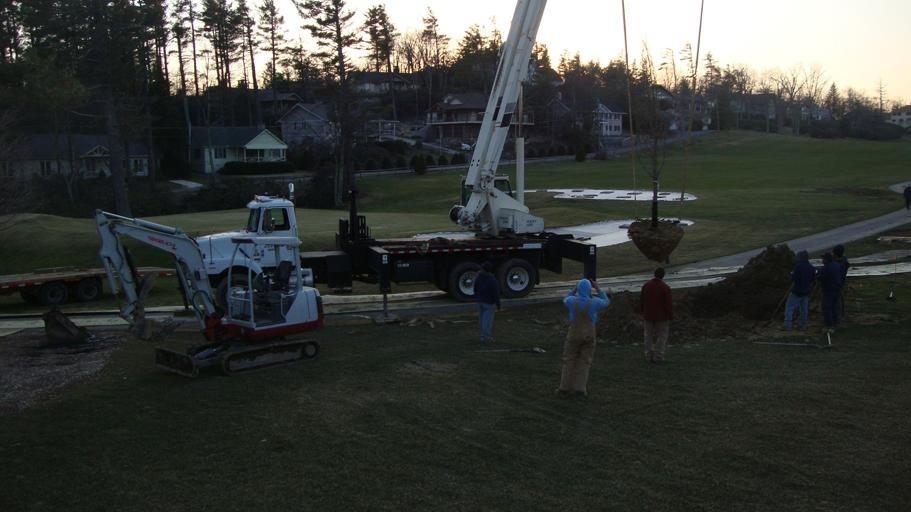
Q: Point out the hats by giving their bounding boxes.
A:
[832,245,843,256]
[797,250,808,261]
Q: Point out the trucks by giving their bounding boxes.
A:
[0,266,173,305]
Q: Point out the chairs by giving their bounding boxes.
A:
[258,260,292,299]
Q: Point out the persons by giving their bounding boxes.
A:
[775,250,816,332]
[817,244,850,333]
[556,278,611,398]
[639,266,673,362]
[903,183,911,210]
[474,263,500,344]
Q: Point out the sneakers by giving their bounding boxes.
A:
[481,336,495,344]
[821,327,834,335]
[554,387,588,398]
[777,323,807,331]
